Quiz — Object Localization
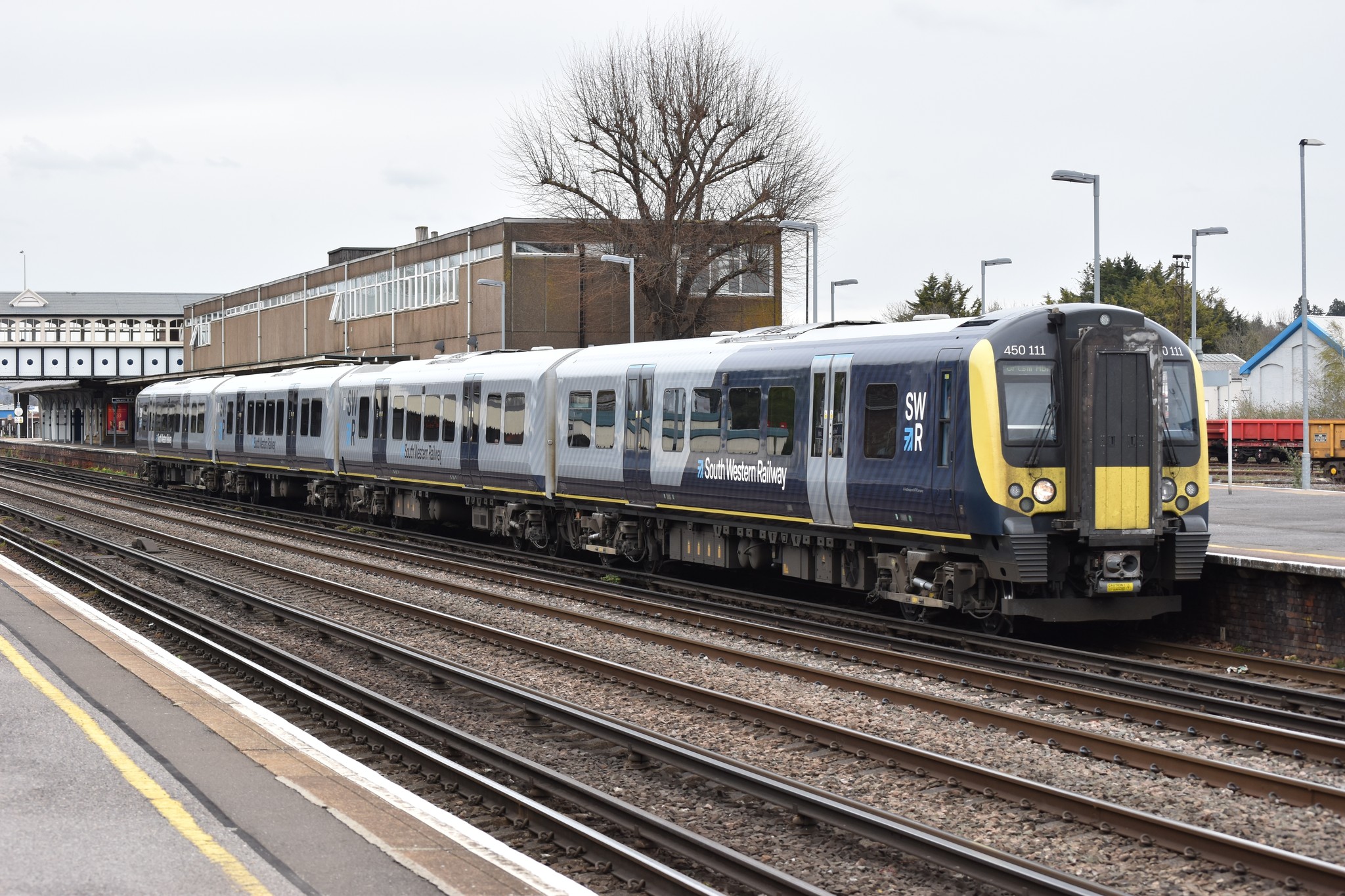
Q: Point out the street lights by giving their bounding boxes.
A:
[14,407,23,438]
[601,254,635,343]
[477,278,507,349]
[981,258,1012,314]
[1191,226,1230,364]
[20,250,25,291]
[1300,138,1326,489]
[830,279,859,321]
[1172,254,1192,342]
[1049,170,1101,302]
[778,219,817,325]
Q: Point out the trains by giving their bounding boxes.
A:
[1205,419,1303,464]
[135,303,1213,634]
[1307,421,1345,483]
